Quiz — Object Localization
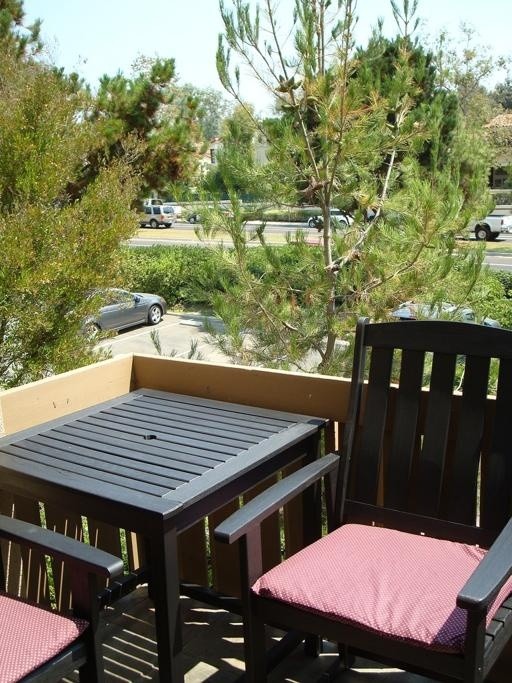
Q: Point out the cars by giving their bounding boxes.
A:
[308,208,356,227]
[375,301,501,364]
[187,208,232,224]
[66,287,167,341]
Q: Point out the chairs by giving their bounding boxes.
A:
[0,516,125,682]
[213,319,510,683]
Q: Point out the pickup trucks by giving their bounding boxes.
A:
[466,215,512,241]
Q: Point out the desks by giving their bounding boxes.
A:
[0,385,328,682]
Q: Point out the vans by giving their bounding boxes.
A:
[138,199,175,228]
